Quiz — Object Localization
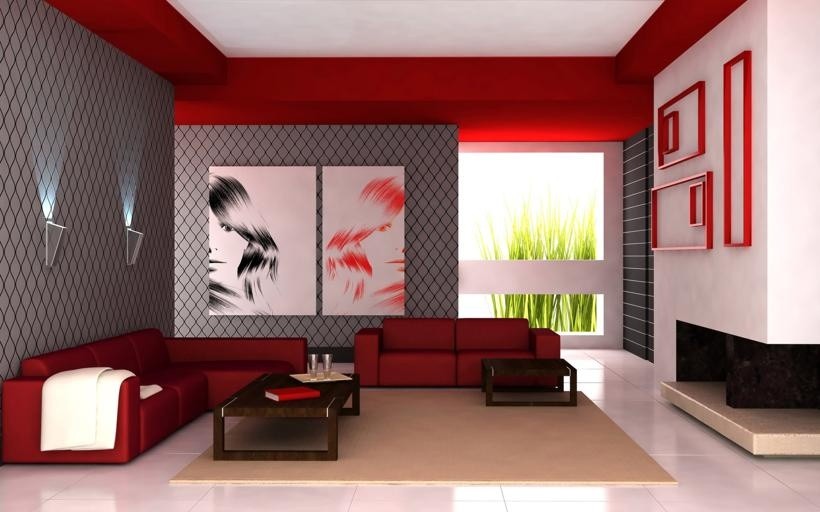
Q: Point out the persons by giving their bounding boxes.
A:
[210,174,282,316]
[326,178,404,313]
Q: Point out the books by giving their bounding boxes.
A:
[264,386,321,402]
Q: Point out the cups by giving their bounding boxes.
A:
[308,354,319,378]
[322,353,334,378]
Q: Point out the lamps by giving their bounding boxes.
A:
[169,391,678,484]
[46,221,67,266]
[126,227,143,265]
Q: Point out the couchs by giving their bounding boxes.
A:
[1,326,307,465]
[354,318,560,388]
